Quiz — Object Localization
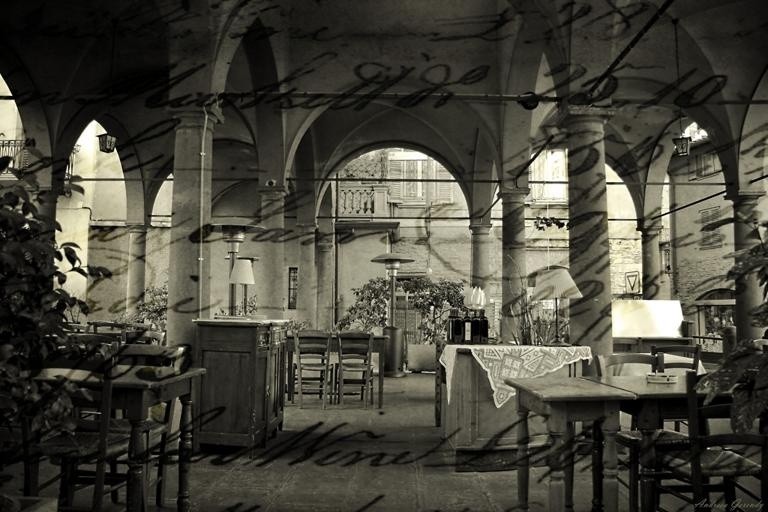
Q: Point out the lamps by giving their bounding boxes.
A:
[95,126,117,154]
[530,267,584,348]
[670,11,692,159]
[230,257,255,318]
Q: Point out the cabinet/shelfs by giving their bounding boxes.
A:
[441,344,596,475]
[433,338,450,426]
[188,314,293,461]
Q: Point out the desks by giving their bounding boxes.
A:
[505,376,636,512]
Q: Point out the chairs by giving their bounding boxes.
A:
[290,326,332,414]
[597,343,767,512]
[2,321,208,512]
[331,330,376,407]
